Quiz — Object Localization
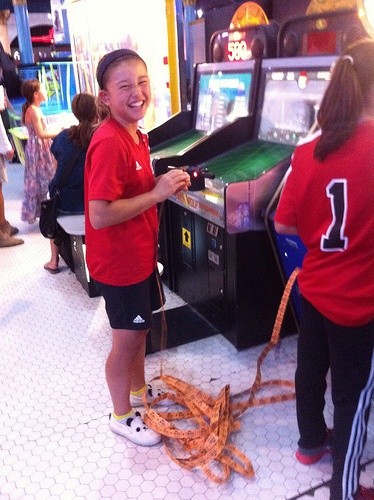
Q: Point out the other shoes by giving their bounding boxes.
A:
[0,220,19,236]
[0,230,24,247]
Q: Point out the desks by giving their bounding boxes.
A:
[9,126,29,166]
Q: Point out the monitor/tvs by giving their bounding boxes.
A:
[194,68,254,132]
[255,67,332,147]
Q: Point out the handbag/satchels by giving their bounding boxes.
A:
[39,199,59,239]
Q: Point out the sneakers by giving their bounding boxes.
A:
[130,384,178,407]
[355,486,374,500]
[295,428,333,465]
[107,407,162,445]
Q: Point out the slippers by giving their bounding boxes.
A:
[44,262,60,274]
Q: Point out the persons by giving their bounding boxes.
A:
[274,40,374,500]
[0,94,23,247]
[21,80,59,223]
[84,48,192,445]
[43,93,97,273]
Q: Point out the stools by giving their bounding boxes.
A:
[57,216,101,298]
